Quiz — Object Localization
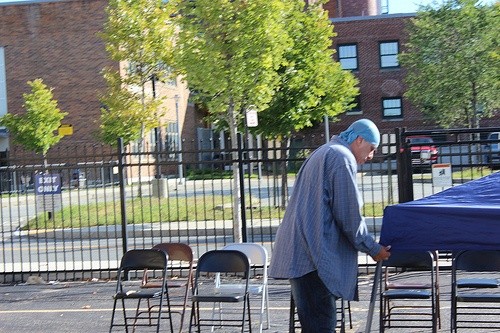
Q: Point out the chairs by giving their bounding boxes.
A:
[110,243,270,333]
[380,249,441,333]
[451,250,500,333]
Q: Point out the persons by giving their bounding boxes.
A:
[266,118,391,333]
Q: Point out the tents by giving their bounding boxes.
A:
[365,171,500,333]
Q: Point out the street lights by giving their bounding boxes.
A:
[149,72,163,179]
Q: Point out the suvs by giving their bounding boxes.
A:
[401,136,439,174]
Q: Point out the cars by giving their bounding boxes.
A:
[484,130,499,168]
